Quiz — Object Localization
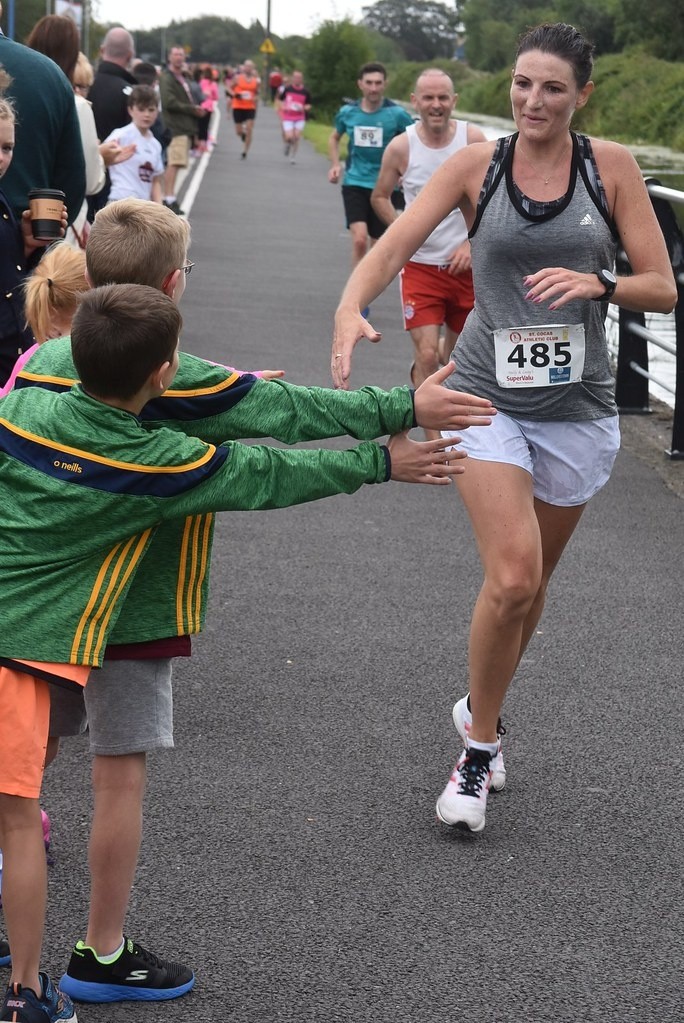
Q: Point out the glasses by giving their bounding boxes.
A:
[163,258,195,289]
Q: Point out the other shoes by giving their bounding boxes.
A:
[40,807,49,858]
[360,307,369,320]
[284,144,292,157]
[163,200,185,215]
[0,939,11,966]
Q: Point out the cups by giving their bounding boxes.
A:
[29,188,65,240]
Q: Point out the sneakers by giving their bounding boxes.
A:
[1,972,78,1023]
[435,740,499,832]
[452,690,506,793]
[59,936,195,1001]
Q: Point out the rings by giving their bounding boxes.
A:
[335,354,341,359]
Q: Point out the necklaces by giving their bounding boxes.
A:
[512,139,573,184]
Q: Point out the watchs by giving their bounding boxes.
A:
[591,269,619,304]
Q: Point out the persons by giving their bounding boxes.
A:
[69,53,109,242]
[0,197,499,1004]
[371,66,491,476]
[278,70,312,165]
[330,23,677,834]
[26,14,107,241]
[0,62,68,385]
[0,283,469,1023]
[0,234,287,850]
[328,62,414,325]
[158,45,205,215]
[129,42,288,166]
[345,89,397,169]
[89,27,171,226]
[0,0,86,347]
[101,82,166,208]
[224,59,261,159]
